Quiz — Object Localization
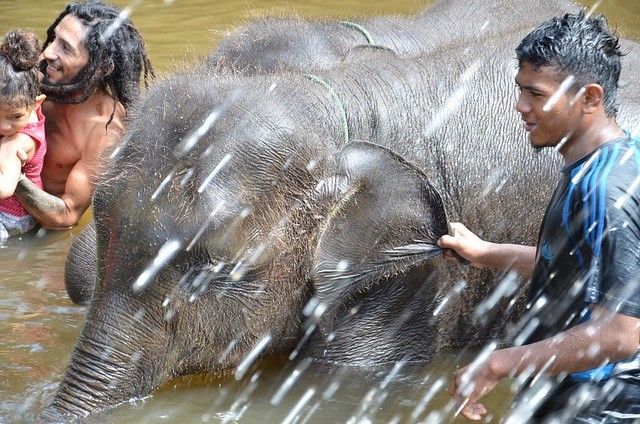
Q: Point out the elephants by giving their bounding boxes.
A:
[63,1,589,309]
[35,22,640,424]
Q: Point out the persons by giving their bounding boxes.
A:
[437,9,640,424]
[0,28,47,244]
[13,1,156,230]
